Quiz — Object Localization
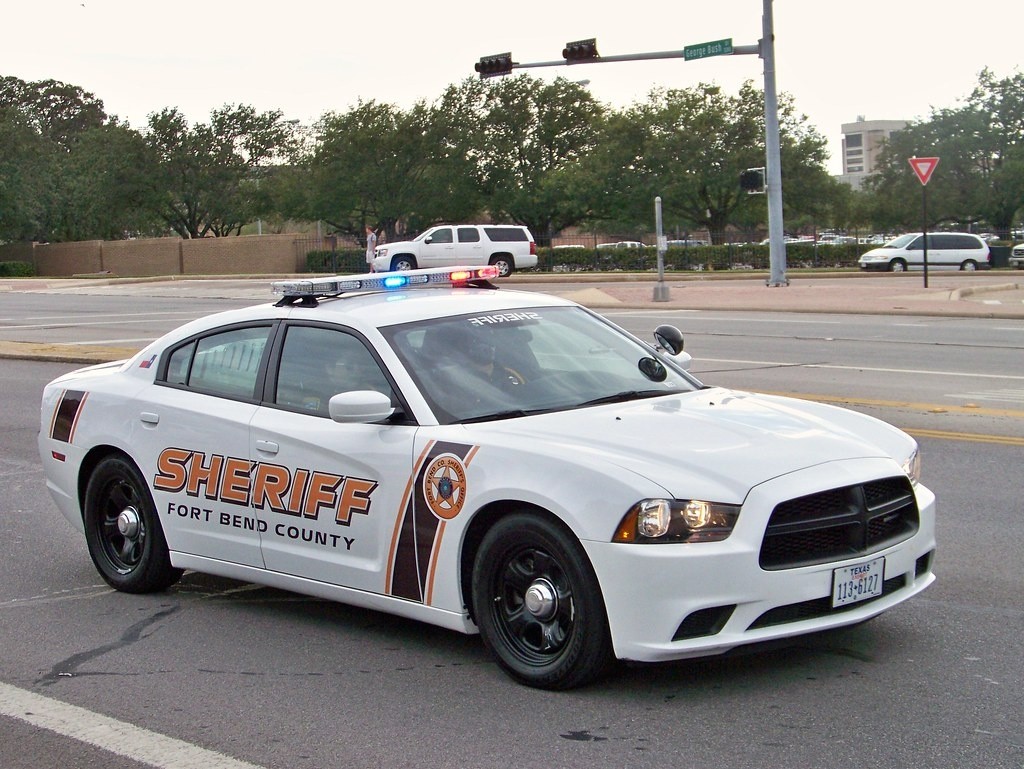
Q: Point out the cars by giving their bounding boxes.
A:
[551,228,904,247]
[1009,240,1024,268]
[38,265,939,692]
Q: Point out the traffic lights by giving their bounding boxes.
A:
[564,38,598,63]
[475,54,513,75]
[739,169,766,194]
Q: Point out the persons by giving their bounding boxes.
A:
[366,226,378,275]
[292,344,389,417]
[458,323,531,410]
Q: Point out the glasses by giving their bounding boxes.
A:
[330,361,368,372]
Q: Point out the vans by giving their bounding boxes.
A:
[857,232,992,274]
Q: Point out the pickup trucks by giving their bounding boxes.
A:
[372,223,540,278]
